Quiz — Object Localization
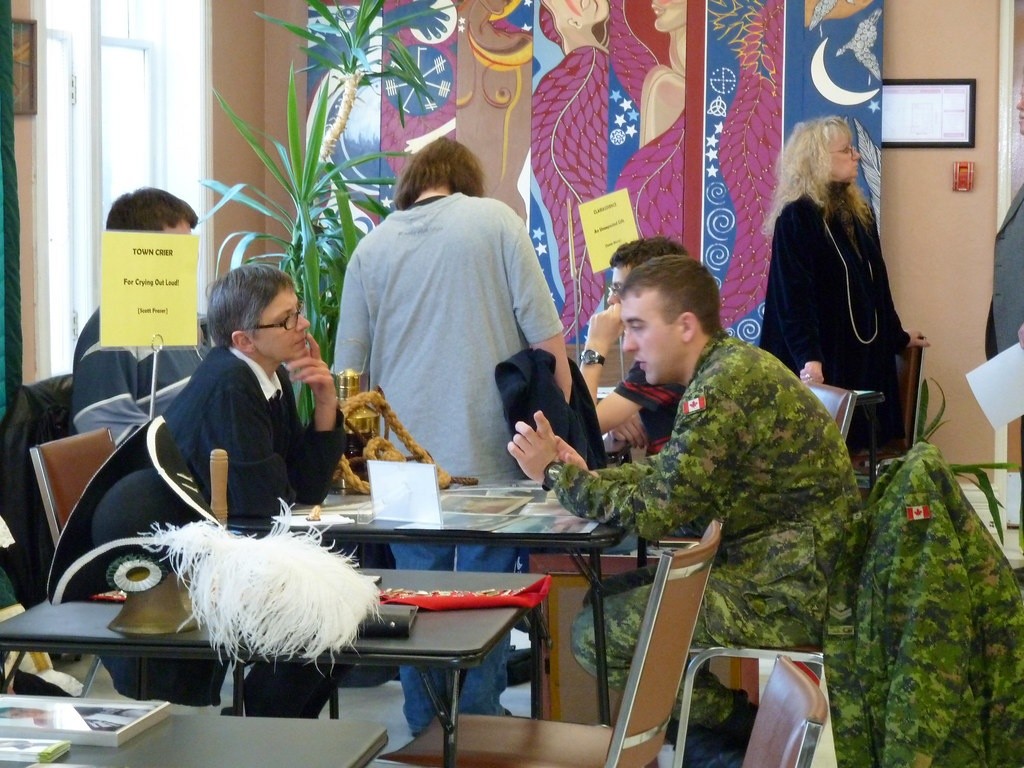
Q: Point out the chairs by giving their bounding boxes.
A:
[32,427,342,719]
[372,336,1024,768]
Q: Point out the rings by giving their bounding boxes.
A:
[806,374,810,379]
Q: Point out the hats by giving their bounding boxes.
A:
[46,416,384,667]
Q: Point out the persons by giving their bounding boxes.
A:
[0,706,53,727]
[332,134,572,739]
[507,254,865,768]
[155,265,350,719]
[577,237,700,539]
[71,187,216,444]
[758,116,930,448]
[984,75,1024,590]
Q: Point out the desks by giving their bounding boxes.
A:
[0,569,553,767]
[268,477,631,728]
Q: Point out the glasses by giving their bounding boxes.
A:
[229,303,306,343]
[830,145,856,156]
[608,286,623,297]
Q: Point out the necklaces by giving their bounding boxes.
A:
[808,186,879,345]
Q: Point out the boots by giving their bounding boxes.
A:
[673,689,759,768]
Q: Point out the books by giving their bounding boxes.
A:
[0,696,171,748]
[354,604,418,639]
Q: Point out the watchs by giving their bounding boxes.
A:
[542,461,564,491]
[580,349,605,366]
